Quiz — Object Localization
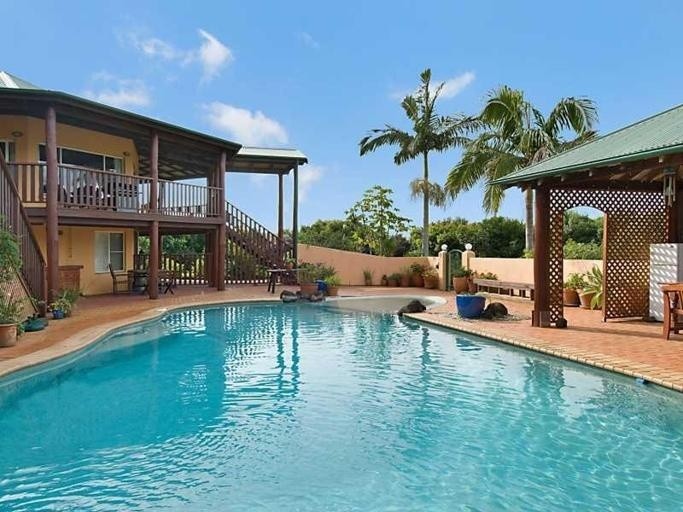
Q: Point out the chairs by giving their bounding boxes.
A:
[108,263,133,295]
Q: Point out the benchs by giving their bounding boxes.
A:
[473,278,535,301]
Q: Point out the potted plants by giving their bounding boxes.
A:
[299,260,343,297]
[362,263,439,289]
[564,264,604,310]
[46,287,88,319]
[0,213,40,348]
[284,257,296,269]
[450,265,497,320]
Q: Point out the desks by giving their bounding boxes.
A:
[128,270,179,296]
[268,268,304,293]
